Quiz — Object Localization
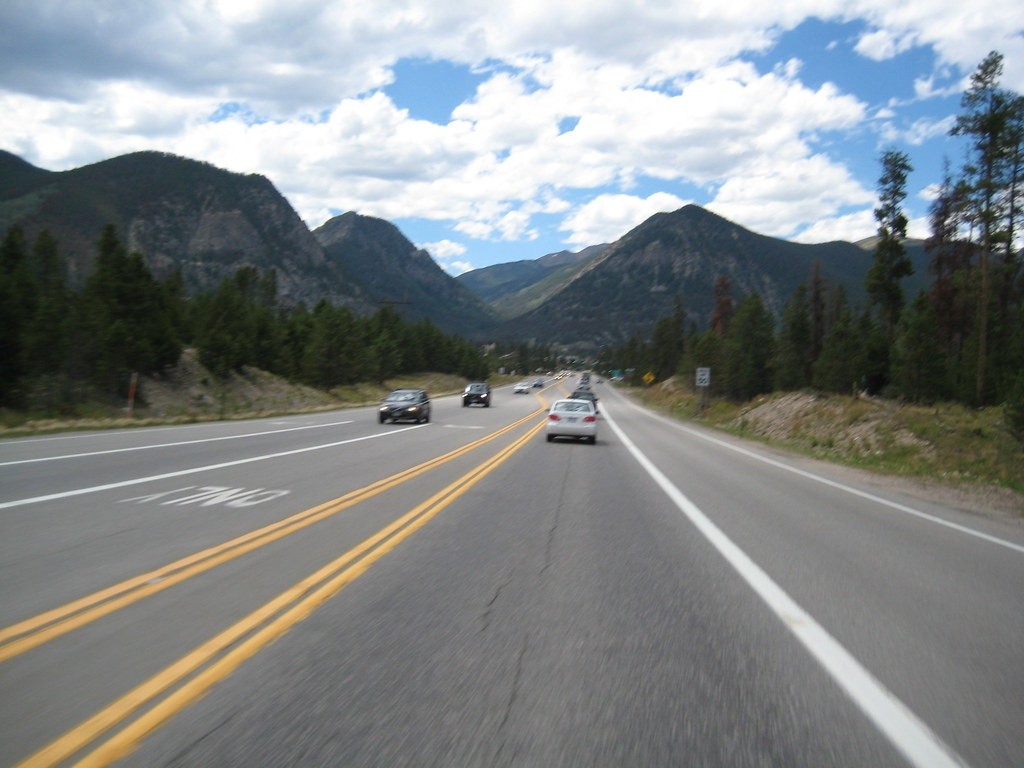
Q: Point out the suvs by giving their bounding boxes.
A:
[462,383,491,406]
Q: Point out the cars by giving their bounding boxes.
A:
[513,382,530,394]
[378,387,431,424]
[545,370,600,444]
